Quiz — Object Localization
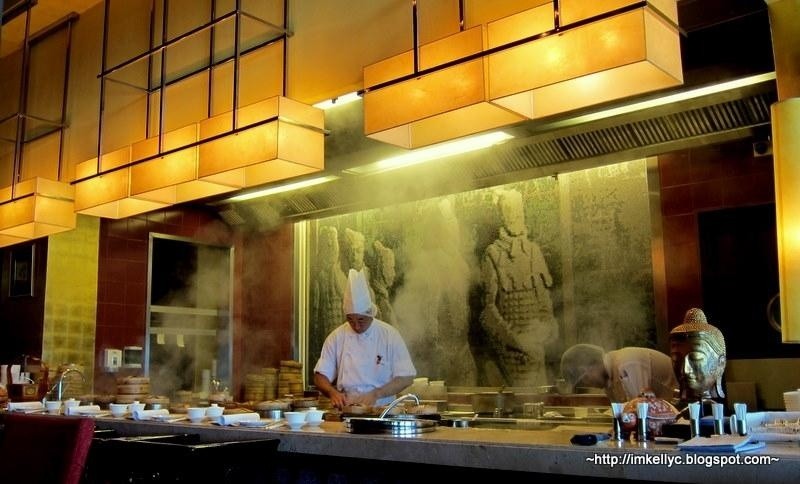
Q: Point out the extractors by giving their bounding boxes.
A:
[215,83,772,237]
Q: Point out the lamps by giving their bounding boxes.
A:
[70,0,331,219]
[358,1,688,150]
[0,0,81,248]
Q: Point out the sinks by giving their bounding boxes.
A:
[341,414,437,435]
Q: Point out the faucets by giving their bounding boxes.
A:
[58,368,86,400]
[378,393,420,420]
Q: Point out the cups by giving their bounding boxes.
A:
[44,397,327,430]
[714,419,724,436]
[612,417,624,441]
[736,420,747,436]
[690,418,701,438]
[636,418,650,442]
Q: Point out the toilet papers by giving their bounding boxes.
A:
[64,405,100,416]
[133,409,169,420]
[219,412,260,426]
[7,401,43,412]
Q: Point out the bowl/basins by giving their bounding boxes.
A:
[556,380,576,395]
[413,376,446,400]
[467,392,516,414]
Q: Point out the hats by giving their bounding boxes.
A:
[343,268,378,318]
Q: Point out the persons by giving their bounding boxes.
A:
[668,307,728,403]
[313,310,417,411]
[315,189,560,388]
[561,343,680,406]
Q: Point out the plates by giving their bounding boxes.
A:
[782,391,800,411]
